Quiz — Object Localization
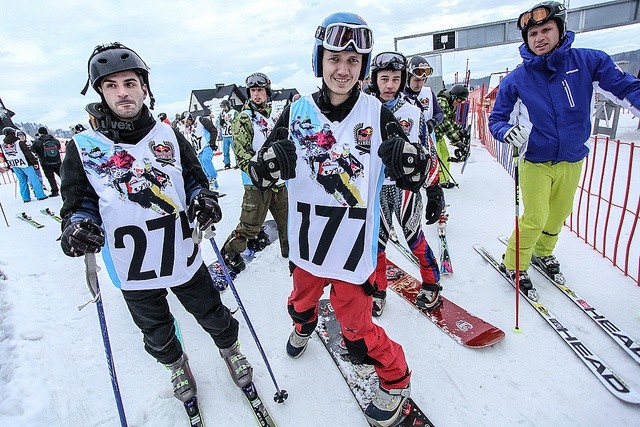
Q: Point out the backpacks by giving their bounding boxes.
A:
[39,138,60,163]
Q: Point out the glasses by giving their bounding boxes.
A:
[369,52,407,71]
[220,103,228,108]
[406,67,433,78]
[18,133,25,135]
[451,94,467,103]
[246,74,271,87]
[518,4,566,32]
[315,23,374,54]
[181,112,189,124]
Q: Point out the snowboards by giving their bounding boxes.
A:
[315,299,434,427]
[386,257,505,348]
[207,220,280,291]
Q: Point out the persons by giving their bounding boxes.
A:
[58,42,253,403]
[389,56,449,243]
[157,112,171,125]
[215,100,240,169]
[0,127,48,202]
[172,113,179,127]
[434,84,471,188]
[368,51,445,317]
[181,111,219,191]
[32,127,63,197]
[65,124,86,145]
[220,72,289,273]
[247,11,433,426]
[487,2,640,295]
[15,129,47,196]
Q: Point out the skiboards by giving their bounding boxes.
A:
[388,229,453,276]
[173,317,277,427]
[16,210,62,228]
[471,232,639,404]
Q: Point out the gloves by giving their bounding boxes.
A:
[378,137,433,185]
[460,144,467,153]
[426,185,444,224]
[426,120,434,134]
[211,145,218,150]
[189,194,222,231]
[61,221,105,257]
[504,125,531,148]
[220,118,225,126]
[247,140,297,190]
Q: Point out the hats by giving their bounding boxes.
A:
[39,127,47,134]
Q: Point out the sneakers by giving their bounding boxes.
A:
[165,352,196,402]
[219,339,253,389]
[39,196,48,200]
[220,246,245,273]
[441,182,454,188]
[287,316,318,359]
[49,192,58,197]
[372,297,386,317]
[209,178,218,190]
[536,255,558,269]
[225,164,230,169]
[24,198,31,202]
[365,380,411,427]
[502,254,530,283]
[416,284,440,309]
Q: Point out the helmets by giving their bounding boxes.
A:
[221,100,230,108]
[81,42,157,110]
[245,73,272,103]
[3,127,16,136]
[312,12,372,81]
[522,1,568,56]
[450,85,468,103]
[408,56,431,80]
[181,112,192,121]
[371,52,408,92]
